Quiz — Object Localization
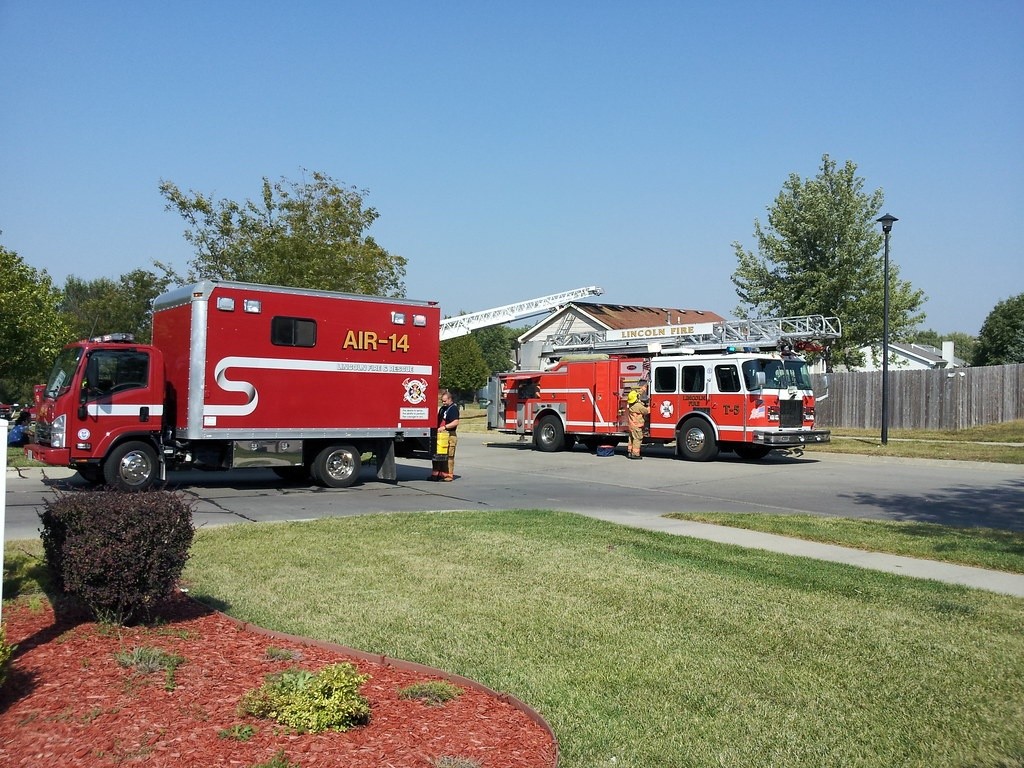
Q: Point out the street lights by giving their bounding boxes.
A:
[877,212,900,446]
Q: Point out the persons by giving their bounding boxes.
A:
[60,379,88,391]
[7,409,35,448]
[627,384,651,459]
[427,391,459,482]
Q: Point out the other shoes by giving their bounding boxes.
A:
[441,476,452,481]
[426,475,440,482]
[628,453,632,457]
[631,453,642,459]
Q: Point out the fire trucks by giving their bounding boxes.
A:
[485,313,842,462]
[21,279,604,494]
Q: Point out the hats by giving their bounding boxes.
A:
[627,390,639,403]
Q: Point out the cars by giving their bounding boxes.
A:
[0,401,11,420]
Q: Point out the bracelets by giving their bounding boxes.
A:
[443,425,447,430]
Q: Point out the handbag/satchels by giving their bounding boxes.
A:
[597,445,614,456]
